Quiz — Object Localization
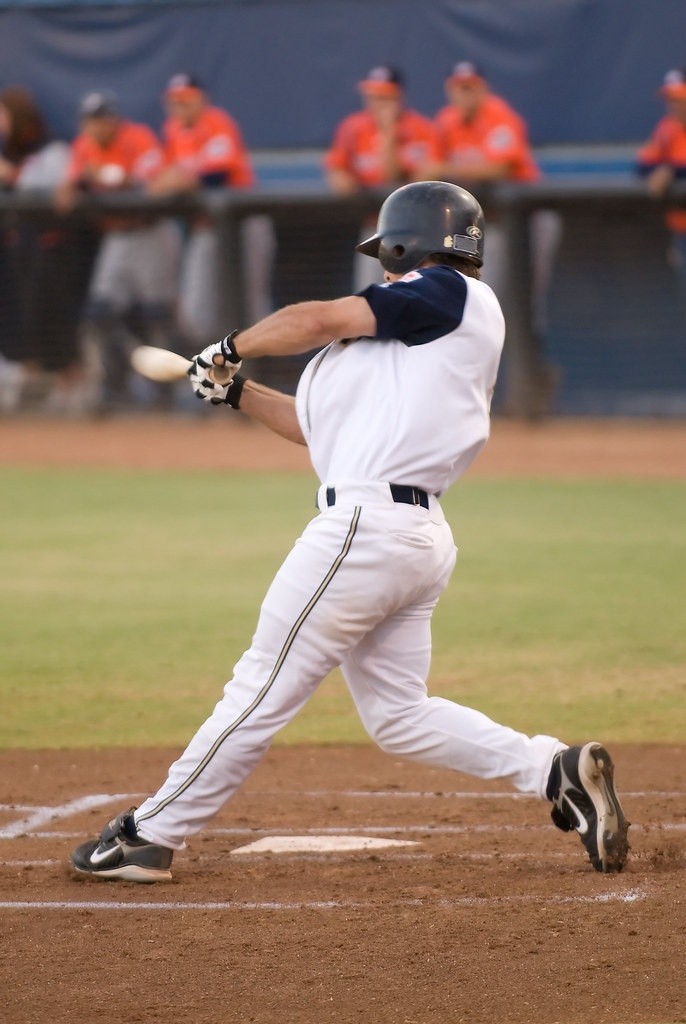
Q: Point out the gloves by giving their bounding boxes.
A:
[187,329,248,410]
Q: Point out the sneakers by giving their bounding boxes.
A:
[71,805,174,882]
[550,742,628,873]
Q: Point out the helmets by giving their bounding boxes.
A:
[355,181,485,274]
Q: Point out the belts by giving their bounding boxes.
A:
[315,485,429,509]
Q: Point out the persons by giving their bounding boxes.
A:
[0,58,686,418]
[70,180,629,882]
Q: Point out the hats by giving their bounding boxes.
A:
[359,67,403,98]
[78,88,117,118]
[445,60,484,92]
[163,73,203,105]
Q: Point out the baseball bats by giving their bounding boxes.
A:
[129,344,235,387]
